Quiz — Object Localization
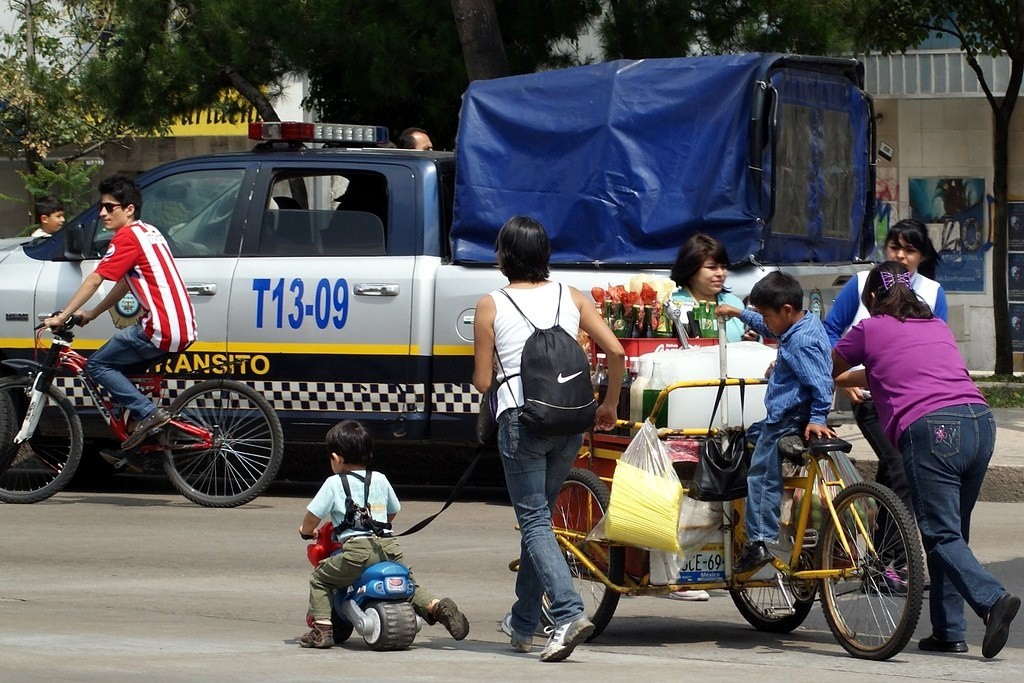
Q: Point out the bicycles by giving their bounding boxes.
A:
[0,309,308,507]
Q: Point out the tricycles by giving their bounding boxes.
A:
[508,370,929,661]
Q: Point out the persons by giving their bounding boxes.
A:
[299,420,470,648]
[399,127,433,151]
[472,215,625,662]
[822,218,939,593]
[667,233,763,600]
[831,261,1021,659]
[31,196,65,237]
[714,270,834,573]
[44,176,198,473]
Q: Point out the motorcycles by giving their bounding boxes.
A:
[299,520,422,651]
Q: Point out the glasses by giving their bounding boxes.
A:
[98,202,129,213]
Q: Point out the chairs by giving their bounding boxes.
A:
[318,200,387,250]
[258,196,319,256]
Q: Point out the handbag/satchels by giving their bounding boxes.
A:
[478,383,501,442]
[792,442,879,541]
[688,429,749,501]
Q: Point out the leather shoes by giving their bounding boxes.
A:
[122,409,171,450]
[736,543,771,570]
[982,593,1019,659]
[919,632,969,651]
[97,449,146,475]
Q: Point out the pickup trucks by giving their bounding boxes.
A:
[0,56,880,503]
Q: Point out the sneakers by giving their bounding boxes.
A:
[652,590,710,600]
[501,612,534,652]
[540,615,596,661]
[861,564,907,594]
[897,567,931,590]
[298,626,335,647]
[434,598,470,640]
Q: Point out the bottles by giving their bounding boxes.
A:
[594,299,718,339]
[586,352,669,441]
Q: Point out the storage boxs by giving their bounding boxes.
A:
[590,337,718,371]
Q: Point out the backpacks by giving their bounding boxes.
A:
[495,283,598,431]
